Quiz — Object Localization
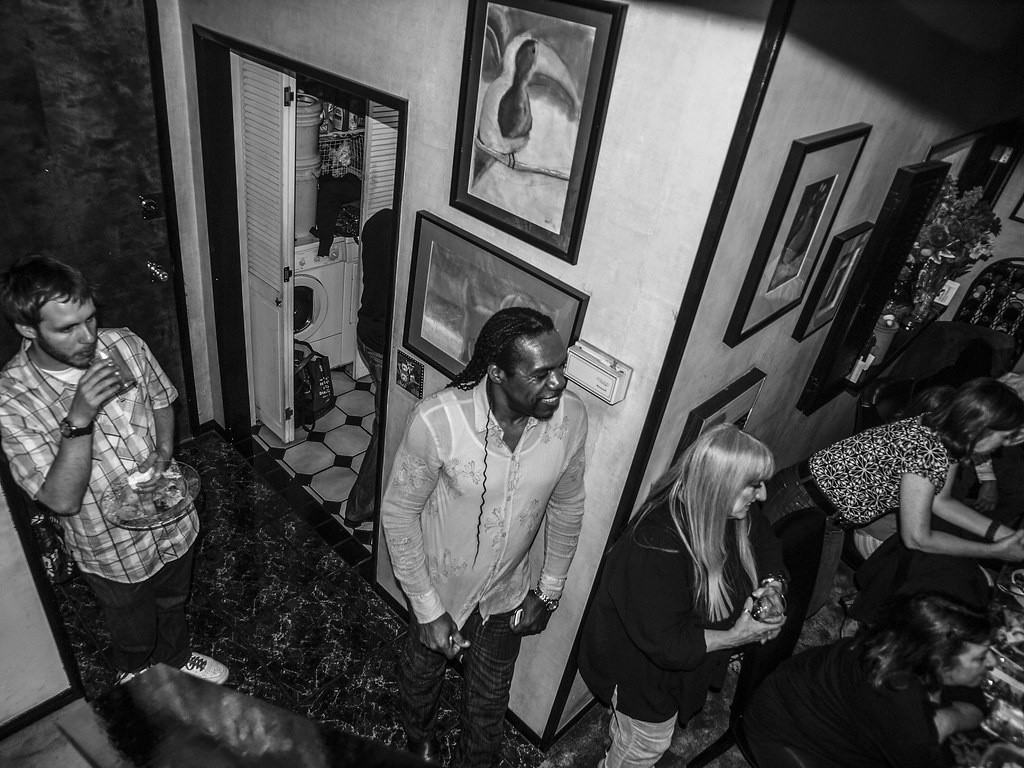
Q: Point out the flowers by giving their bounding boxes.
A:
[916,174,1002,296]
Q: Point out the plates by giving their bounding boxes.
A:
[977,568,1024,768]
[99,460,200,529]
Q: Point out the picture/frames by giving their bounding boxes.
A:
[722,122,874,350]
[924,132,981,182]
[670,367,767,469]
[401,209,589,402]
[449,0,630,266]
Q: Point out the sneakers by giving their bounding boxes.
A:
[115,665,154,686]
[180,651,230,686]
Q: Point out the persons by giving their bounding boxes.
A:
[854,386,955,559]
[971,372,1024,513]
[381,307,585,767]
[1,254,229,685]
[342,208,394,528]
[728,377,1024,674]
[742,587,998,768]
[578,421,787,767]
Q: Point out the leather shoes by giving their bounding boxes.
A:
[407,735,442,768]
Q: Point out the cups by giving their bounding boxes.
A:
[750,588,786,622]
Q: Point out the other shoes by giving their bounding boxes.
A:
[841,616,861,638]
[344,513,375,528]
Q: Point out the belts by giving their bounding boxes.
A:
[798,456,853,531]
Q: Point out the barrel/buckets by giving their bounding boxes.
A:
[296,92,322,239]
[869,315,898,367]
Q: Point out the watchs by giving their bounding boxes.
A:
[534,588,559,612]
[59,418,94,440]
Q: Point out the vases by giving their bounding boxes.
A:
[913,289,935,322]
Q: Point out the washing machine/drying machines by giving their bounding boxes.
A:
[292,233,345,370]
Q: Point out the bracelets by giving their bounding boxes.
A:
[985,521,1000,541]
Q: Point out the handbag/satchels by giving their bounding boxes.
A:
[294,338,335,432]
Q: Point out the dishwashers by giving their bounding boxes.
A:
[294,237,346,368]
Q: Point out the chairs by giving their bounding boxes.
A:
[685,505,826,768]
